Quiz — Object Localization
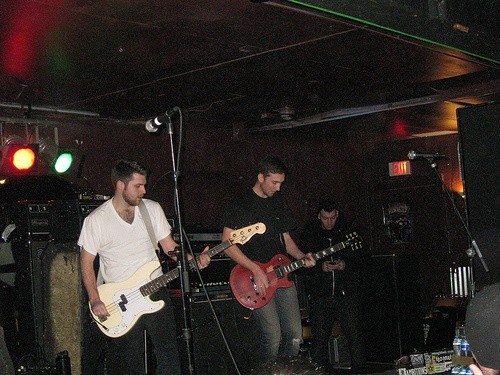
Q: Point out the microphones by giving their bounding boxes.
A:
[407,150,450,161]
[146,106,179,132]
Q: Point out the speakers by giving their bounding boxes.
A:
[186,287,251,375]
[12,239,110,374]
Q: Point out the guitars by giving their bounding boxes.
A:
[89,222,267,337]
[229,232,364,310]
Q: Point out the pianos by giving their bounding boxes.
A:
[167,281,230,297]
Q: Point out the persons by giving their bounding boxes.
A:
[249,354,329,375]
[222,156,315,361]
[465,283,500,375]
[295,200,370,375]
[77,159,210,375]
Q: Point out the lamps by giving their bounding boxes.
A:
[0,121,36,170]
[32,125,74,173]
